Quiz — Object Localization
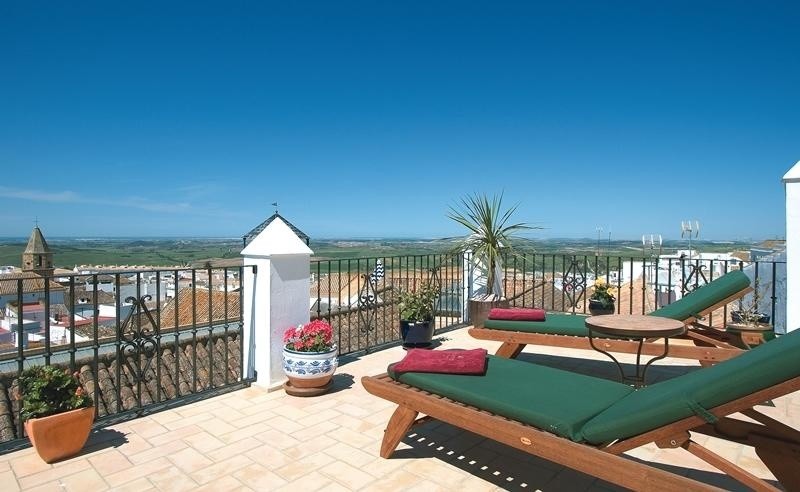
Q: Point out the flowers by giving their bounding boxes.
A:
[592,279,614,302]
[14,367,93,420]
[282,320,334,351]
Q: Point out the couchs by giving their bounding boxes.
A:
[361,328,800,492]
[468,269,768,369]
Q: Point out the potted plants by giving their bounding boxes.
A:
[443,190,552,328]
[398,282,435,351]
[726,277,776,349]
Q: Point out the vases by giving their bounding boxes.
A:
[282,345,339,396]
[23,407,95,464]
[730,310,770,324]
[588,297,615,316]
